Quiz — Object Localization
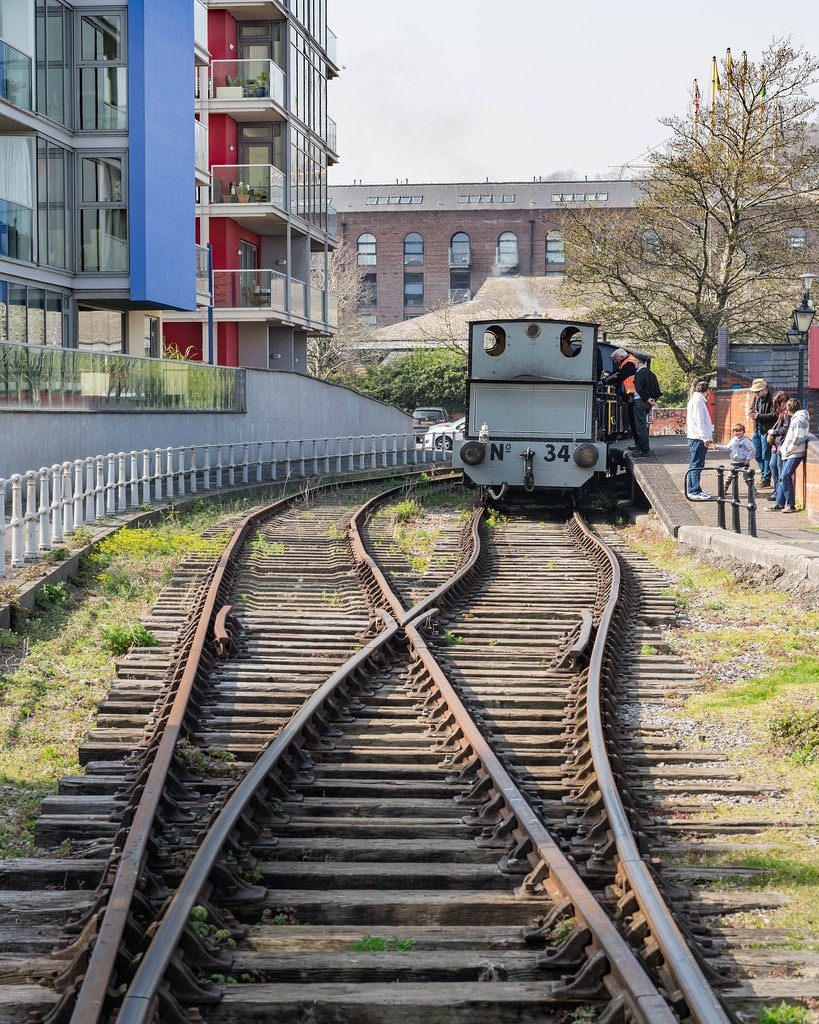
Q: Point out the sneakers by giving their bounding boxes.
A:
[687,493,693,497]
[633,450,649,457]
[693,492,712,499]
[627,445,640,450]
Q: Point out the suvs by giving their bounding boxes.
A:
[413,406,450,443]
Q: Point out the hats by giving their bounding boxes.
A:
[750,378,768,391]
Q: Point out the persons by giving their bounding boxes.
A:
[631,354,663,458]
[596,370,612,435]
[598,349,639,449]
[714,423,758,495]
[687,382,713,499]
[748,378,772,488]
[764,391,810,513]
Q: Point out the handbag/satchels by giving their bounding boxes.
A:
[767,433,773,444]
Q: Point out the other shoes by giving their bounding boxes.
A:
[768,505,783,511]
[781,506,796,512]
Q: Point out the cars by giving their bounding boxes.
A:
[424,417,466,449]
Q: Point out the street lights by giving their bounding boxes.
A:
[798,273,818,301]
[791,292,818,412]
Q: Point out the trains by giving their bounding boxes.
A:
[451,318,656,518]
